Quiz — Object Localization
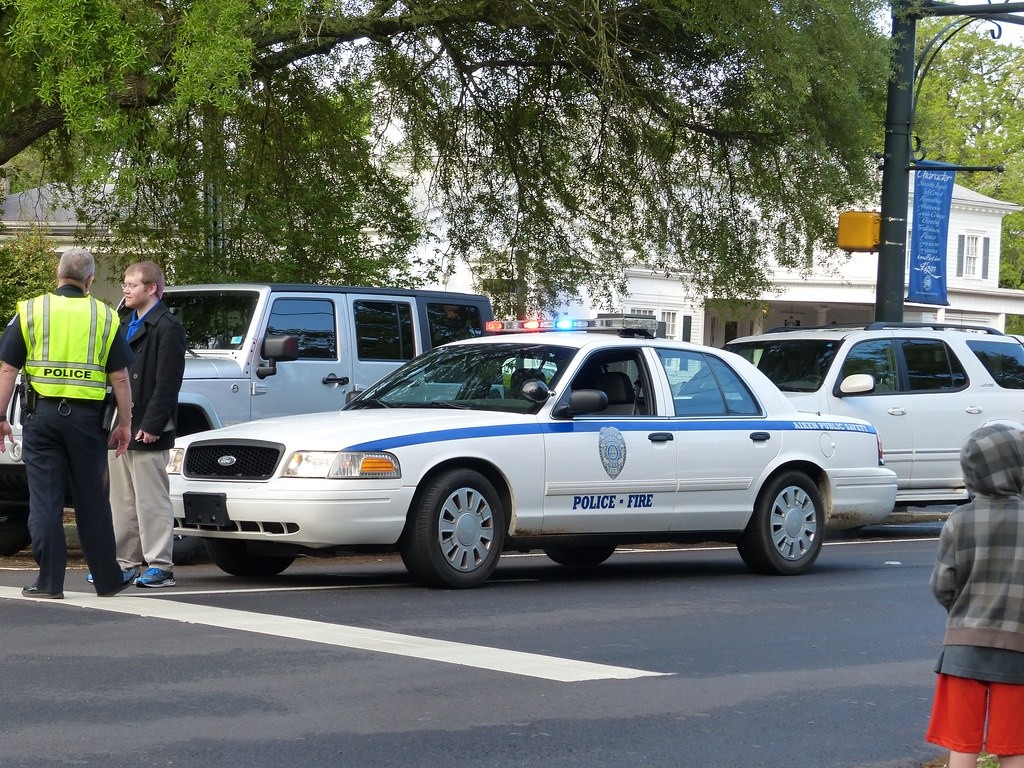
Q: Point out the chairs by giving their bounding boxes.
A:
[506,368,642,415]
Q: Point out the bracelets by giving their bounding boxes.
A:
[0,415,7,422]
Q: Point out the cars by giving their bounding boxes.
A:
[0,284,504,557]
[166,318,898,590]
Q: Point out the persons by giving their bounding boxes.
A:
[926,424,1024,768]
[0,249,136,599]
[86,260,187,588]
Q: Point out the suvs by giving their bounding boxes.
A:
[673,322,1024,507]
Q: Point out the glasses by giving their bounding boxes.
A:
[121,283,148,290]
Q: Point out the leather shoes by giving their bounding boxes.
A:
[98,569,135,597]
[21,585,64,599]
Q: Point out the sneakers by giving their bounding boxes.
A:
[136,568,176,588]
[87,570,141,583]
